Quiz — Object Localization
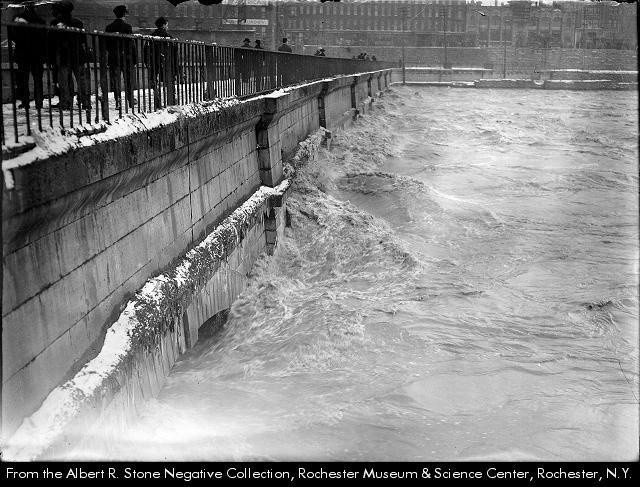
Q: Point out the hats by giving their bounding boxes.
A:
[255,39,261,44]
[281,37,288,41]
[113,4,129,17]
[155,16,169,26]
[50,0,74,11]
[243,37,251,43]
[316,48,326,53]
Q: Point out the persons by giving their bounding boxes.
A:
[277,37,292,52]
[252,39,266,82]
[8,0,48,109]
[103,5,138,110]
[48,0,93,110]
[319,48,326,56]
[240,37,253,82]
[314,49,321,56]
[351,51,377,60]
[143,14,180,110]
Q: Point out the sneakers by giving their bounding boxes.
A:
[115,101,138,109]
[52,102,92,111]
[17,103,31,110]
